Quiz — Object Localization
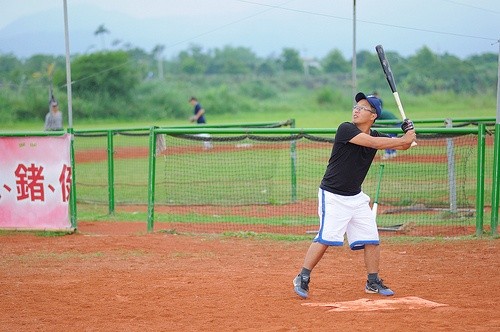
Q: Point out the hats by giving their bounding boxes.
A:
[355,92,383,119]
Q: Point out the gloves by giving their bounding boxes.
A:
[401,119,414,134]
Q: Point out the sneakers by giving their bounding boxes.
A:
[366,279,394,296]
[293,274,310,298]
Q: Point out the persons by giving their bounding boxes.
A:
[189,97,211,150]
[374,109,402,160]
[292,89,417,298]
[43,99,63,131]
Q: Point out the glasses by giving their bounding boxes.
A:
[354,105,374,114]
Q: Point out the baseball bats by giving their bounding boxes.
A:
[371,163,385,221]
[375,45,417,148]
